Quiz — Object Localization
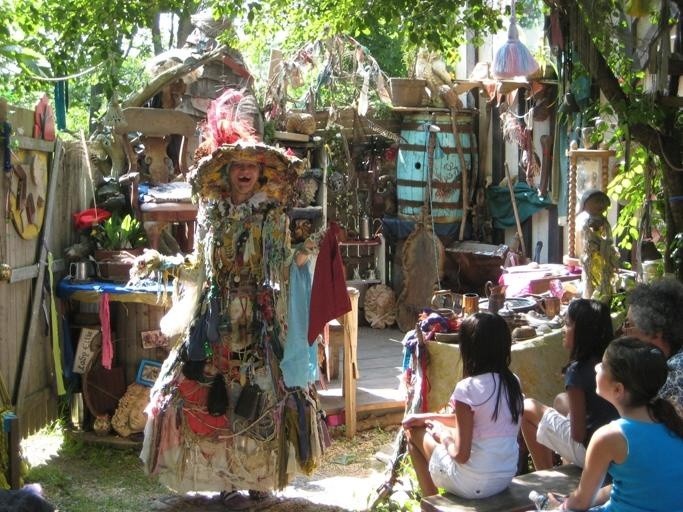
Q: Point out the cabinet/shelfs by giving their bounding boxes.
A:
[337,232,385,285]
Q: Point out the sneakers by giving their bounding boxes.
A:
[220,490,251,510]
[249,489,268,498]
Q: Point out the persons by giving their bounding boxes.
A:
[618,273,682,421]
[524,332,682,511]
[128,157,332,510]
[399,309,525,512]
[517,298,615,475]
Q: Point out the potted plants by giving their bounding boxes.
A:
[91,214,146,281]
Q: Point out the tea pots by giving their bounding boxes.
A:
[486,281,508,313]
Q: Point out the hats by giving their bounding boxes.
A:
[186,138,300,203]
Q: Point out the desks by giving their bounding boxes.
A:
[402,311,626,413]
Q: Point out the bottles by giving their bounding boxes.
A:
[528,489,553,512]
[201,341,214,363]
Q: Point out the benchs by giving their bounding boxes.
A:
[420,463,611,510]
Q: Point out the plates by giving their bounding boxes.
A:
[478,297,537,311]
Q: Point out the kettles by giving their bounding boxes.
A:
[69,256,96,284]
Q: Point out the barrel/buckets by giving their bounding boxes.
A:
[397,113,479,226]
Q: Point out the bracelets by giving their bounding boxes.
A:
[557,500,569,511]
[298,244,314,256]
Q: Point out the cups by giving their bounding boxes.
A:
[541,297,561,318]
[462,293,479,315]
